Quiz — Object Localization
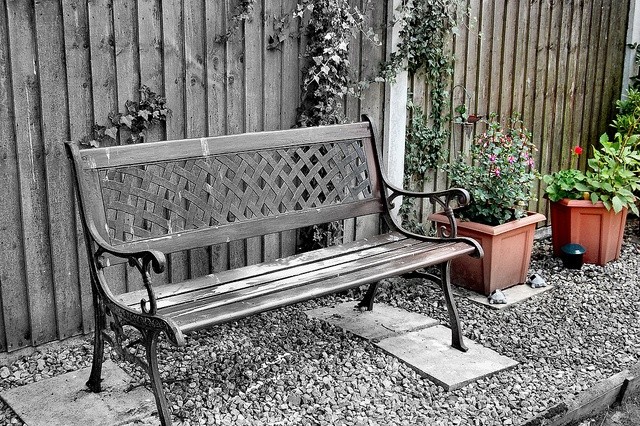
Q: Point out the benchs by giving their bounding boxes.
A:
[60,110,485,425]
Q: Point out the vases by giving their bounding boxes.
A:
[427,203,549,296]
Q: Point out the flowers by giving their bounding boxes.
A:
[436,111,545,225]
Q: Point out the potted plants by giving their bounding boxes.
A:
[542,86,639,267]
